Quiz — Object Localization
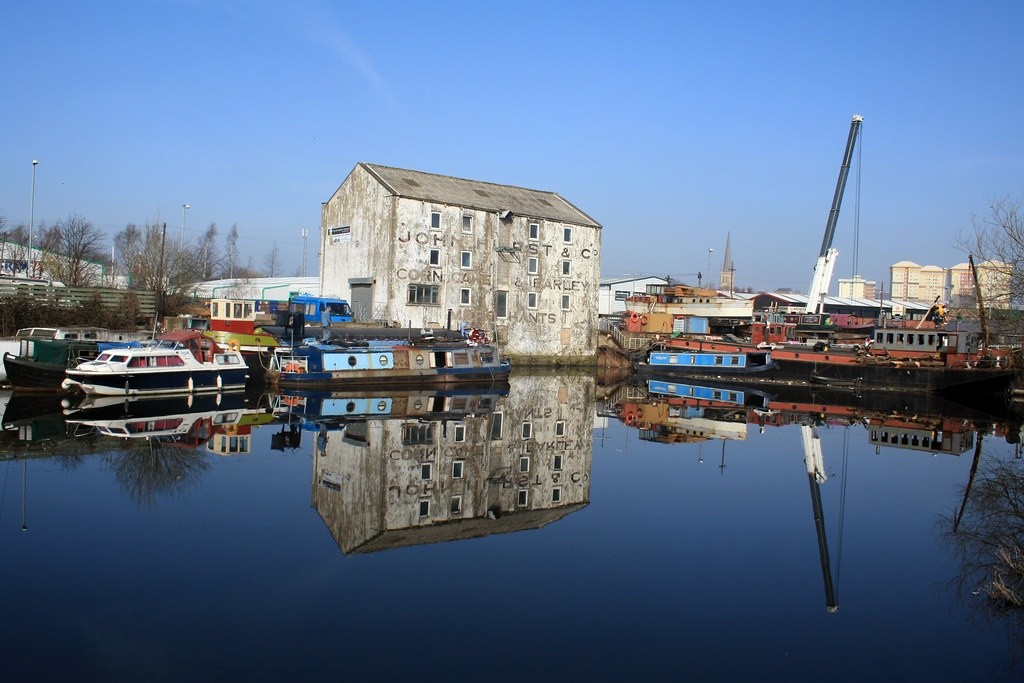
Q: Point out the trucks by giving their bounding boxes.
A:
[291,296,356,322]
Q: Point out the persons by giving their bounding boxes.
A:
[317,422,329,456]
[320,306,332,342]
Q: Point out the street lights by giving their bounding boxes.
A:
[179,204,191,282]
[28,160,39,276]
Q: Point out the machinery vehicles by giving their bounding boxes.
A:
[781,114,865,334]
[793,412,849,614]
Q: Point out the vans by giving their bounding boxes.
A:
[15,280,82,308]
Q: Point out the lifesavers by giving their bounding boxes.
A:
[626,411,634,422]
[636,408,645,419]
[286,364,300,373]
[224,424,239,436]
[228,339,240,351]
[630,312,640,323]
[641,315,649,325]
[285,395,301,406]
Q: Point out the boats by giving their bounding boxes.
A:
[253,382,511,452]
[2,337,144,396]
[194,297,295,367]
[63,311,250,397]
[61,389,249,468]
[1,387,94,446]
[255,311,511,383]
